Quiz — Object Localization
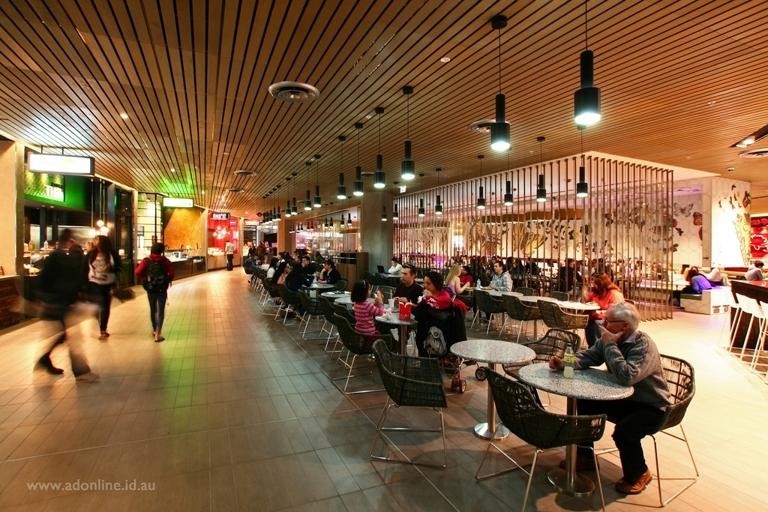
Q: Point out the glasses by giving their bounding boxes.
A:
[604,317,628,324]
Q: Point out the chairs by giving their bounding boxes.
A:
[240,248,768,510]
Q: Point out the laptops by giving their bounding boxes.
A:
[377,265,392,274]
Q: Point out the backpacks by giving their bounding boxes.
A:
[142,256,170,293]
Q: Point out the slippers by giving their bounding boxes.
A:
[152,330,166,343]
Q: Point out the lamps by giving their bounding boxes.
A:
[256,1,615,236]
[735,135,756,149]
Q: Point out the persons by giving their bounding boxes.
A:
[670,269,712,307]
[134,243,176,344]
[27,227,99,382]
[516,256,664,295]
[244,240,516,366]
[745,260,765,281]
[84,235,130,340]
[580,273,626,349]
[690,266,699,272]
[224,240,235,270]
[548,301,672,495]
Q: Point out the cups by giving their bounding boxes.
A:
[388,298,396,310]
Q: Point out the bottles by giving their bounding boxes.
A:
[476,277,482,289]
[564,344,574,378]
[44,239,49,249]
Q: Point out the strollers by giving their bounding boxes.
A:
[411,301,490,397]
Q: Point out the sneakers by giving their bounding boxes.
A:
[74,371,98,383]
[614,467,654,495]
[558,454,601,471]
[35,354,66,375]
